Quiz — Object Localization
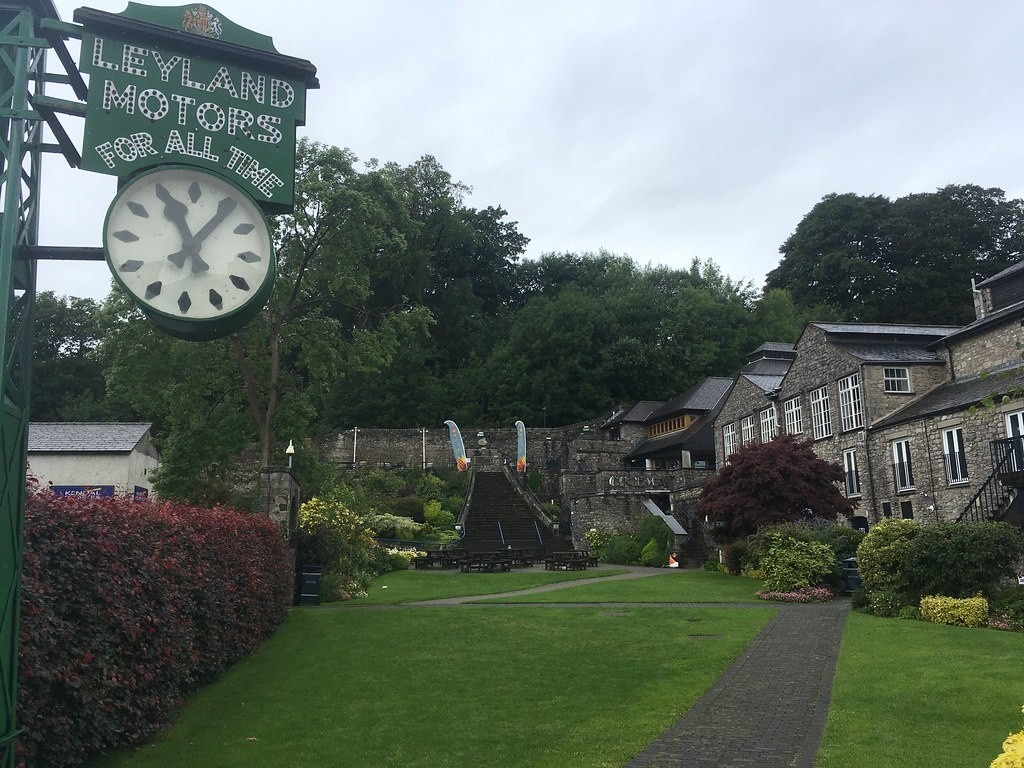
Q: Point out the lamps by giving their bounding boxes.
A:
[553,521,559,529]
[455,523,462,530]
[285,439,295,467]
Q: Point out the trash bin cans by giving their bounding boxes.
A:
[670,549,682,567]
[297,564,323,605]
[842,557,863,597]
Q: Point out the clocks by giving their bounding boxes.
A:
[102,165,276,342]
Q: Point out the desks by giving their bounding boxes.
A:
[450,548,472,565]
[424,548,453,570]
[521,547,547,564]
[569,549,594,568]
[471,552,500,571]
[552,552,582,570]
[496,548,526,569]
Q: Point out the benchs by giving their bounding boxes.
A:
[533,553,549,564]
[441,557,460,570]
[413,557,437,569]
[565,558,587,571]
[459,560,490,573]
[516,556,535,569]
[544,559,568,570]
[582,555,602,567]
[491,559,514,574]
[460,555,480,564]
